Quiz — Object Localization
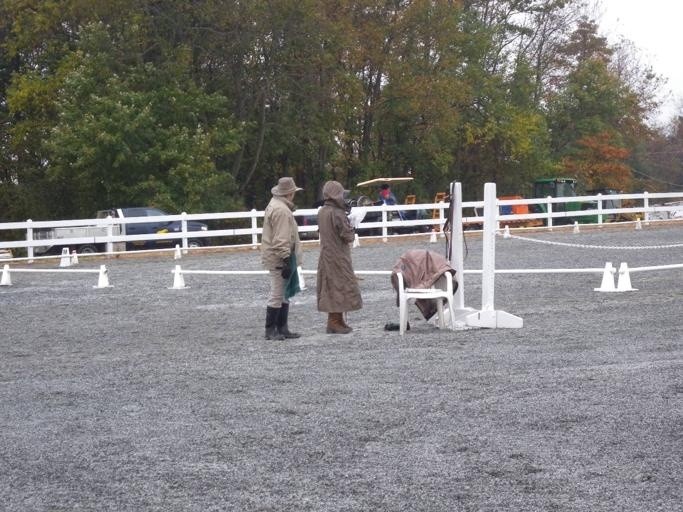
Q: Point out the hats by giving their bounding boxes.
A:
[380,190,389,196]
[270,176,305,196]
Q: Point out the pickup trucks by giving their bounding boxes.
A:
[23,205,212,258]
[291,211,319,241]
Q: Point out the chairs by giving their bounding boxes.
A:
[392,251,457,335]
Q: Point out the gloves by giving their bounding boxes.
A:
[281,258,292,280]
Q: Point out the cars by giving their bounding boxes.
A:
[0,249,13,259]
[396,206,433,235]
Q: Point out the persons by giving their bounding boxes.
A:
[380,184,407,220]
[261,177,304,341]
[316,180,363,334]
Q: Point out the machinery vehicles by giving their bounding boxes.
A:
[493,173,647,230]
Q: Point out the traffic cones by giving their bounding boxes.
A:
[294,265,309,292]
[591,259,617,295]
[348,214,646,250]
[612,260,639,293]
[166,261,192,291]
[0,263,16,289]
[173,243,183,259]
[73,249,80,264]
[91,263,114,290]
[57,246,71,266]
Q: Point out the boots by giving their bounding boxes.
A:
[264,302,302,341]
[327,311,353,334]
